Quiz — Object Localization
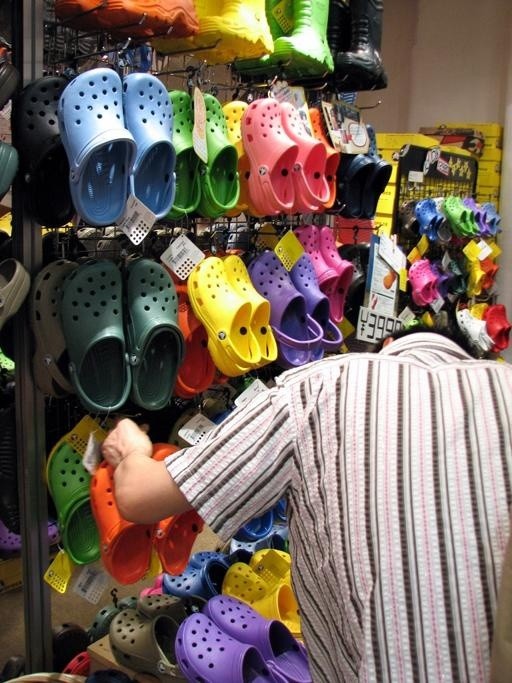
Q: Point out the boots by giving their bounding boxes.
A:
[55,1,387,93]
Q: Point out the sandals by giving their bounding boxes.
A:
[1,433,312,683]
[0,64,392,415]
[407,193,511,356]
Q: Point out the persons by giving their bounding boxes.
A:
[101,325,512,683]
